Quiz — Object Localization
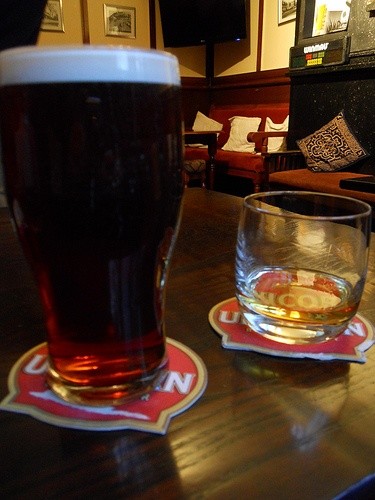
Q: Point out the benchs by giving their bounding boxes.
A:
[260,139,375,235]
[183,103,291,198]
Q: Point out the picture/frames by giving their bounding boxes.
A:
[104,3,137,40]
[40,0,65,33]
[277,0,297,26]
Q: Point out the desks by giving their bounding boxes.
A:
[0,194,375,500]
[182,130,224,191]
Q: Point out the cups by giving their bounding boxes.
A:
[1,42,187,407]
[235,190,372,345]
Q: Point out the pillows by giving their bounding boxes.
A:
[295,108,369,173]
[186,110,224,147]
[220,115,264,151]
[255,114,290,156]
